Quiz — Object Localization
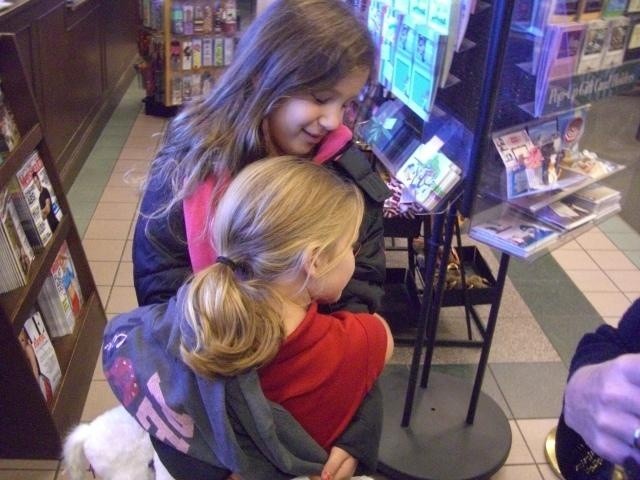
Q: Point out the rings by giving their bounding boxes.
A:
[632,427,640,447]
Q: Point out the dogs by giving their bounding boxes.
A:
[56,404,177,480]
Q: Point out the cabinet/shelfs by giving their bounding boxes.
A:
[377,1,626,480]
[0,32,108,461]
[0,0,141,196]
[139,1,239,117]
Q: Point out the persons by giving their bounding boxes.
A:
[103,155,395,479]
[554,297,640,480]
[130,0,400,480]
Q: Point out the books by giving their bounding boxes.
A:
[360,0,640,259]
[0,85,84,410]
